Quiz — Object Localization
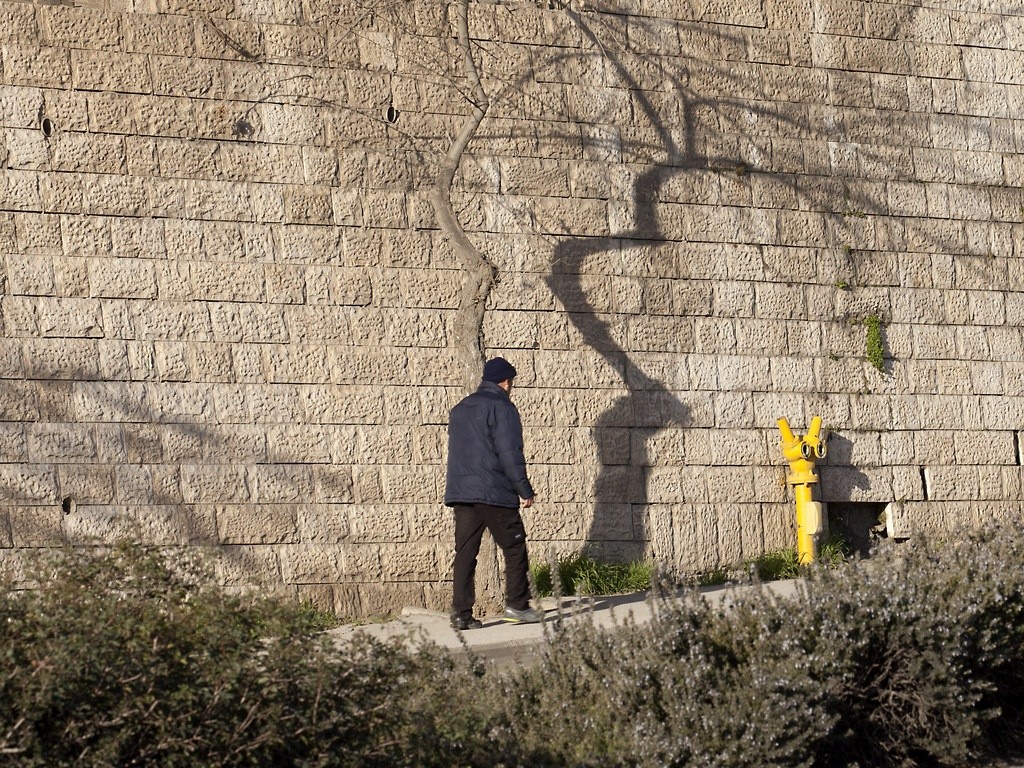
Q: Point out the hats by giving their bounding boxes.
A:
[482,357,517,384]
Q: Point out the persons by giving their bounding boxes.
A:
[445,356,543,630]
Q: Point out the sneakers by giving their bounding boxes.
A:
[449,616,483,630]
[500,607,543,623]
